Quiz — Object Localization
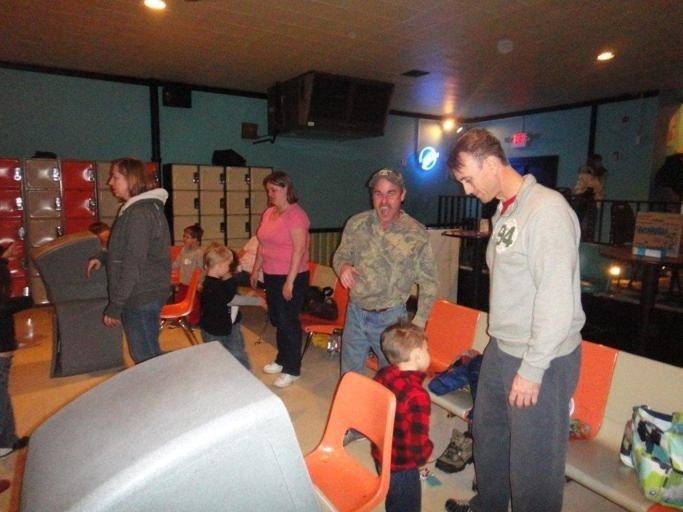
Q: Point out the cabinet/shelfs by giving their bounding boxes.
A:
[1,154,277,306]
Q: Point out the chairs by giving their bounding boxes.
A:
[610,201,636,244]
[578,244,610,297]
[298,370,398,512]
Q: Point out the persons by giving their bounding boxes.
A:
[89,222,112,247]
[333,166,439,448]
[169,222,266,372]
[249,171,311,388]
[0,241,38,459]
[447,127,588,512]
[369,323,436,511]
[574,153,606,243]
[86,158,169,363]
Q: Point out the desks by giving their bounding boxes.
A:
[439,230,490,309]
[595,240,679,355]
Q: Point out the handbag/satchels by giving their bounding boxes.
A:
[298,286,338,321]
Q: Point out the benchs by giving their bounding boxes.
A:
[365,300,683,512]
[156,245,364,370]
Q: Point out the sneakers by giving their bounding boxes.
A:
[168,320,181,328]
[434,429,474,473]
[263,360,284,373]
[273,371,301,388]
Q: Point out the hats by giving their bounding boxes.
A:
[368,169,404,188]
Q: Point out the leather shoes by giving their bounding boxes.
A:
[13,436,28,450]
